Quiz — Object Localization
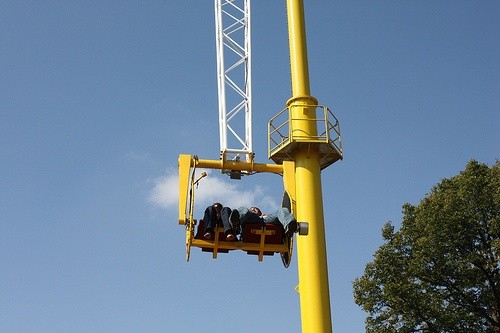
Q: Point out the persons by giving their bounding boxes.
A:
[229,206,297,241]
[203,203,234,241]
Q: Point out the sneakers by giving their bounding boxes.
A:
[284,220,297,237]
